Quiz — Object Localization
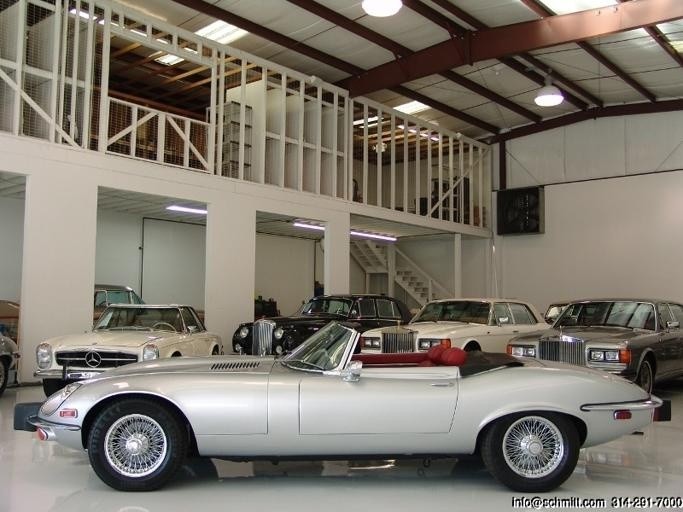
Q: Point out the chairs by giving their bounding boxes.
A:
[417,344,467,368]
[132,311,182,330]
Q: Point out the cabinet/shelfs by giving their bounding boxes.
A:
[205,101,252,183]
[431,163,461,223]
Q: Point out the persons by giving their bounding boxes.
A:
[314,280,323,307]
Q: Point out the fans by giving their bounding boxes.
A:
[496,184,546,237]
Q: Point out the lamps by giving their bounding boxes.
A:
[361,0,404,18]
[532,76,565,109]
